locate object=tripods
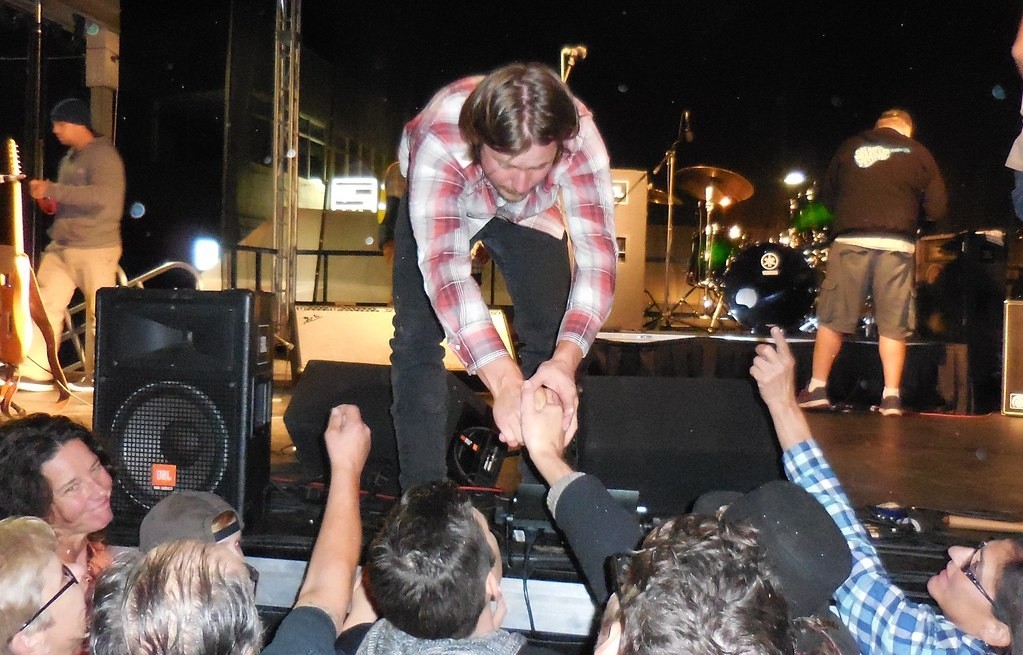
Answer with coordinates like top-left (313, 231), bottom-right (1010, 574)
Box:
top-left (641, 131), bottom-right (742, 337)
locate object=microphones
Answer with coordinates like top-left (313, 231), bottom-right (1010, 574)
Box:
top-left (562, 46), bottom-right (587, 61)
top-left (685, 112), bottom-right (694, 141)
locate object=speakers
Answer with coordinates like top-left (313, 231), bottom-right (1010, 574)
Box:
top-left (915, 231), bottom-right (1006, 415)
top-left (562, 374), bottom-right (787, 520)
top-left (284, 359), bottom-right (509, 498)
top-left (568, 168), bottom-right (647, 331)
top-left (1001, 298), bottom-right (1023, 417)
top-left (92, 287), bottom-right (279, 537)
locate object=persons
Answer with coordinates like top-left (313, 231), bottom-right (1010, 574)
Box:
top-left (0, 412), bottom-right (115, 573)
top-left (936, 336), bottom-right (970, 415)
top-left (520, 381), bottom-right (860, 655)
top-left (0, 515), bottom-right (88, 655)
top-left (334, 482), bottom-right (559, 655)
top-left (138, 488), bottom-right (244, 558)
top-left (593, 514), bottom-right (787, 655)
top-left (750, 327), bottom-right (1023, 655)
top-left (390, 64), bottom-right (618, 491)
top-left (0, 99), bottom-right (124, 392)
top-left (91, 403), bottom-right (371, 655)
top-left (1005, 21), bottom-right (1023, 223)
top-left (798, 110), bottom-right (947, 417)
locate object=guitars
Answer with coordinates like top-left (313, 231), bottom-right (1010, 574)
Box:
top-left (0, 137), bottom-right (30, 367)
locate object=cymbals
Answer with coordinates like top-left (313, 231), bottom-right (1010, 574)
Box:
top-left (647, 187), bottom-right (682, 205)
top-left (673, 166), bottom-right (754, 205)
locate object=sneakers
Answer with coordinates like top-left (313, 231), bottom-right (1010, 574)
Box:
top-left (879, 395), bottom-right (902, 416)
top-left (796, 383), bottom-right (830, 408)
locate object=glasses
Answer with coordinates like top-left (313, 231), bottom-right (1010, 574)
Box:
top-left (610, 552), bottom-right (639, 617)
top-left (959, 534), bottom-right (998, 611)
top-left (5, 564), bottom-right (80, 647)
top-left (243, 563), bottom-right (259, 599)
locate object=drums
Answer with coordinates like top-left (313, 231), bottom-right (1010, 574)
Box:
top-left (685, 224), bottom-right (752, 293)
top-left (723, 243), bottom-right (815, 335)
top-left (789, 187), bottom-right (833, 267)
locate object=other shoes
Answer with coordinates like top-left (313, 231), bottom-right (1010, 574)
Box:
top-left (9, 376), bottom-right (53, 391)
top-left (67, 377), bottom-right (95, 392)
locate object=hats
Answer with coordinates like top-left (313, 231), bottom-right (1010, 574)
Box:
top-left (51, 97), bottom-right (103, 138)
top-left (879, 108), bottom-right (912, 127)
top-left (693, 480), bottom-right (852, 619)
top-left (139, 489), bottom-right (243, 549)
